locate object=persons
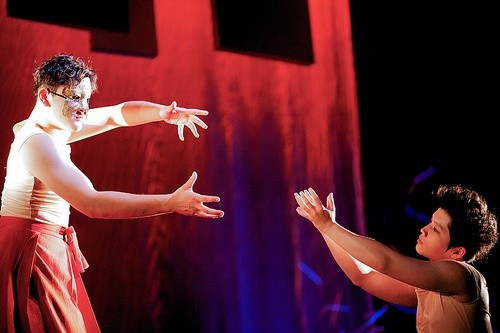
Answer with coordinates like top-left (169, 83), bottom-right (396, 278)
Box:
top-left (0, 52), bottom-right (225, 333)
top-left (295, 183), bottom-right (497, 333)
